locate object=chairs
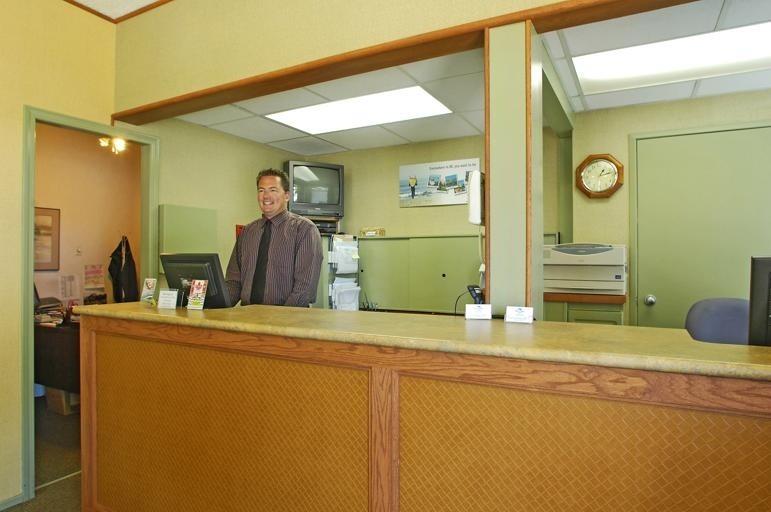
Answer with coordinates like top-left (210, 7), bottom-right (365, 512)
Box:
top-left (685, 296), bottom-right (750, 345)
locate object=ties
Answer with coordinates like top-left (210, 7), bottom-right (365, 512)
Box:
top-left (251, 219), bottom-right (272, 305)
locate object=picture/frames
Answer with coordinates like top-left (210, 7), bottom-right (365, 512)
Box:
top-left (34, 207), bottom-right (61, 272)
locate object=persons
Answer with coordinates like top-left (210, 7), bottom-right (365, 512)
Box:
top-left (225, 169), bottom-right (323, 307)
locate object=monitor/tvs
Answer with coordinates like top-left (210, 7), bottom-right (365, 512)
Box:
top-left (159, 252), bottom-right (231, 308)
top-left (283, 160), bottom-right (345, 217)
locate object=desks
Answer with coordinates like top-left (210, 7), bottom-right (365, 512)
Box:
top-left (34, 322), bottom-right (81, 417)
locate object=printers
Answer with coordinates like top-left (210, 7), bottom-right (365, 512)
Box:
top-left (543, 243), bottom-right (629, 295)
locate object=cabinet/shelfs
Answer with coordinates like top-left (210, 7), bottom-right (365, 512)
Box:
top-left (359, 234), bottom-right (484, 314)
top-left (544, 301), bottom-right (624, 325)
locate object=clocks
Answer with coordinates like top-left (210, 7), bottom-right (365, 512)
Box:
top-left (574, 153), bottom-right (625, 198)
